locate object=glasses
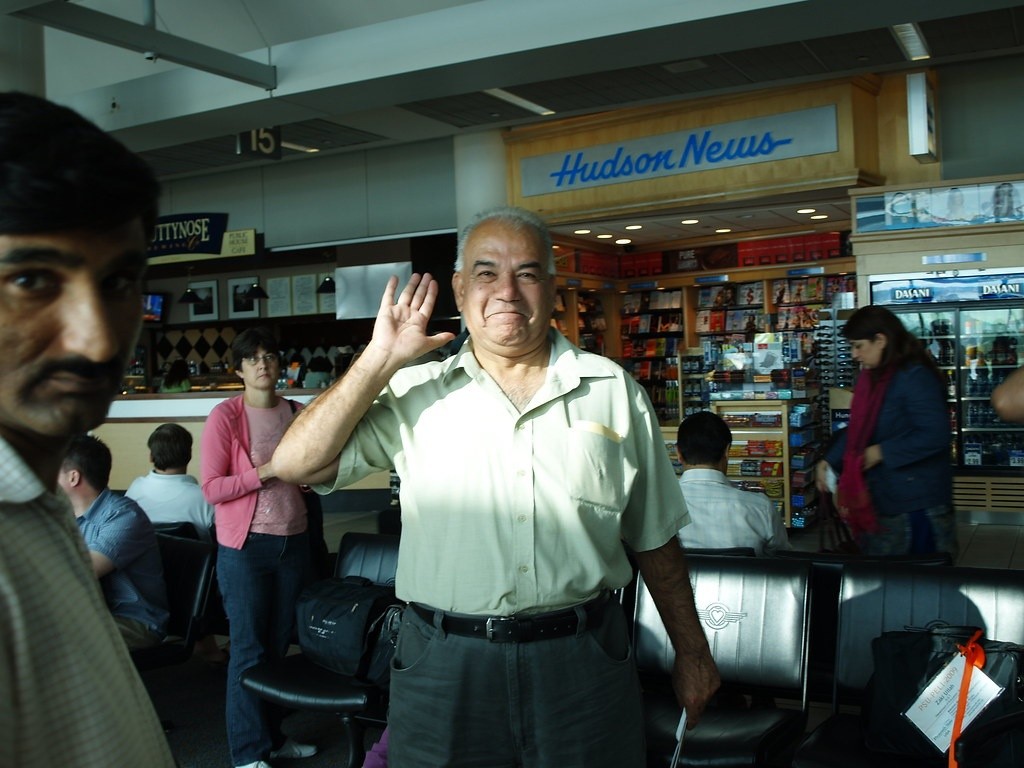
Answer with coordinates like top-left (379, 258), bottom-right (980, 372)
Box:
top-left (242, 353), bottom-right (274, 367)
top-left (814, 321), bottom-right (860, 451)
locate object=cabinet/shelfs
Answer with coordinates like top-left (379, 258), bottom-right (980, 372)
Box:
top-left (615, 282), bottom-right (683, 356)
top-left (552, 276), bottom-right (617, 356)
top-left (684, 272), bottom-right (851, 351)
top-left (707, 400), bottom-right (827, 530)
top-left (677, 345), bottom-right (724, 417)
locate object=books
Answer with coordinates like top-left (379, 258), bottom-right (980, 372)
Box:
top-left (550, 295), bottom-right (607, 351)
top-left (620, 275), bottom-right (857, 380)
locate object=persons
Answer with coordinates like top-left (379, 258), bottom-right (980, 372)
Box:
top-left (271, 206), bottom-right (721, 768)
top-left (124, 423), bottom-right (214, 529)
top-left (675, 410), bottom-right (792, 554)
top-left (795, 285), bottom-right (801, 301)
top-left (928, 319), bottom-right (969, 425)
top-left (783, 308), bottom-right (791, 329)
top-left (304, 356), bottom-right (330, 389)
top-left (159, 358), bottom-right (190, 392)
top-left (816, 303), bottom-right (959, 564)
top-left (59, 434), bottom-right (171, 668)
top-left (745, 328), bottom-right (759, 343)
top-left (776, 287), bottom-right (785, 302)
top-left (0, 91), bottom-right (177, 768)
top-left (745, 315), bottom-right (755, 329)
top-left (201, 330), bottom-right (317, 768)
top-left (991, 363), bottom-right (1024, 423)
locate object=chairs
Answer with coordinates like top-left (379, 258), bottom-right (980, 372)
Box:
top-left (150, 522), bottom-right (1024, 768)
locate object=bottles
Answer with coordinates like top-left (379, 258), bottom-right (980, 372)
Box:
top-left (950, 433), bottom-right (1021, 465)
top-left (781, 333), bottom-right (799, 362)
top-left (947, 369), bottom-right (1009, 428)
top-left (224, 358), bottom-right (229, 372)
top-left (189, 360), bottom-right (197, 375)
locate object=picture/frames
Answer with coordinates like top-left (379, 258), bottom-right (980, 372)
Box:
top-left (188, 280), bottom-right (219, 320)
top-left (228, 274), bottom-right (259, 318)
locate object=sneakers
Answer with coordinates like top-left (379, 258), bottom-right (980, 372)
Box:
top-left (235, 760), bottom-right (272, 768)
top-left (270, 737), bottom-right (317, 759)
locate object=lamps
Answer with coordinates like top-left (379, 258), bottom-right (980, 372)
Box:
top-left (180, 269), bottom-right (205, 304)
top-left (243, 260), bottom-right (268, 299)
top-left (314, 254), bottom-right (337, 294)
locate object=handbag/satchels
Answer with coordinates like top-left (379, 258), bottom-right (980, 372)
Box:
top-left (865, 620), bottom-right (1024, 767)
top-left (818, 489), bottom-right (858, 553)
top-left (296, 575), bottom-right (395, 678)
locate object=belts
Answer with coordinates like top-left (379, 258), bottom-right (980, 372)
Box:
top-left (410, 588), bottom-right (611, 645)
